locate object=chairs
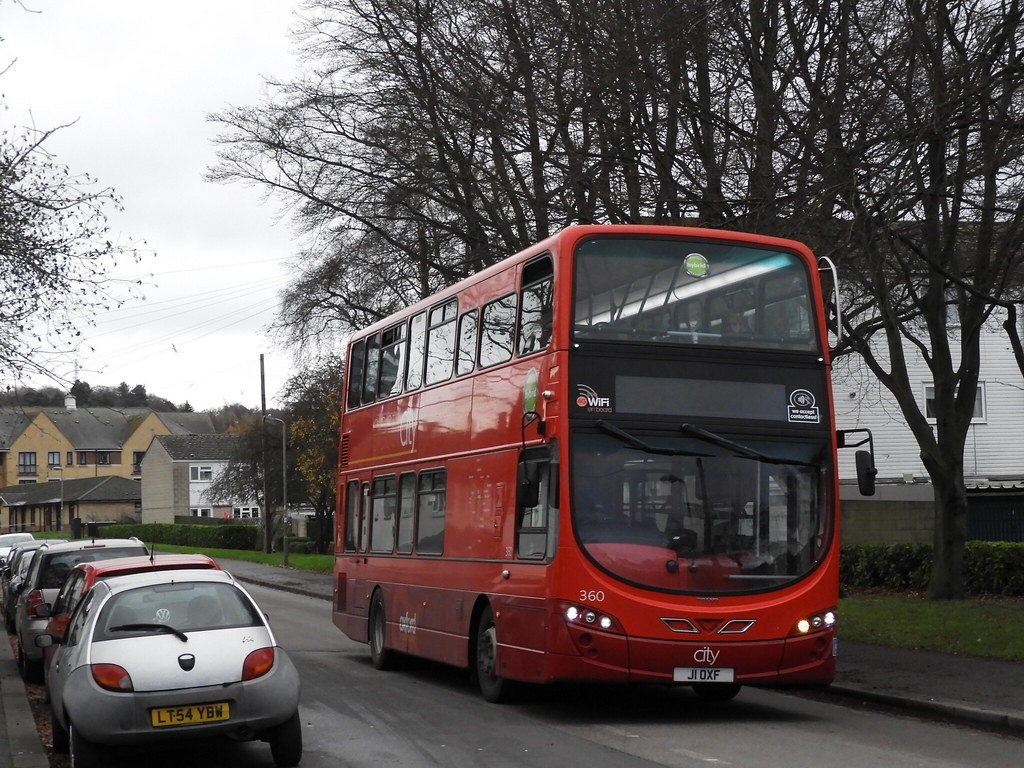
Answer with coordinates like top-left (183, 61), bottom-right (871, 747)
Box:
top-left (182, 596), bottom-right (220, 630)
top-left (109, 606), bottom-right (142, 636)
top-left (43, 562), bottom-right (70, 587)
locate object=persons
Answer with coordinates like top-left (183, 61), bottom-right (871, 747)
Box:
top-left (721, 314), bottom-right (753, 346)
top-left (570, 470), bottom-right (632, 526)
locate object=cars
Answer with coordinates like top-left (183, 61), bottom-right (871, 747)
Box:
top-left (34, 569), bottom-right (302, 768)
top-left (0, 532), bottom-right (222, 709)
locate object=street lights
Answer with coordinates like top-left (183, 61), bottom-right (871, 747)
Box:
top-left (53, 466), bottom-right (64, 539)
top-left (262, 414), bottom-right (289, 567)
